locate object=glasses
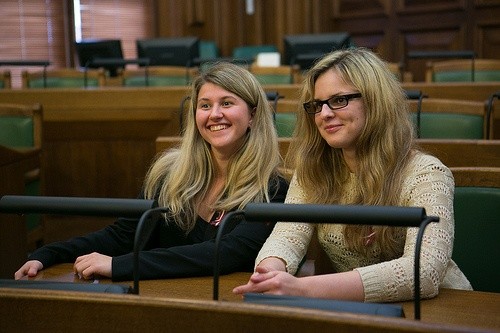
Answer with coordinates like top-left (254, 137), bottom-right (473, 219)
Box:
top-left (303, 93), bottom-right (361, 114)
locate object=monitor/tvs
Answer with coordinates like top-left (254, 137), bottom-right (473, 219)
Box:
top-left (283, 32), bottom-right (352, 73)
top-left (73, 39), bottom-right (126, 77)
top-left (136, 37), bottom-right (200, 69)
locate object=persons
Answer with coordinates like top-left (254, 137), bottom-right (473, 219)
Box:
top-left (15, 61), bottom-right (290, 283)
top-left (233, 46), bottom-right (473, 299)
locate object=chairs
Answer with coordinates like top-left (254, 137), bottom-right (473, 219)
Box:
top-left (0, 44), bottom-right (500, 292)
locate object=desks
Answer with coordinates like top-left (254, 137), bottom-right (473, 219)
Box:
top-left (0, 83), bottom-right (500, 333)
